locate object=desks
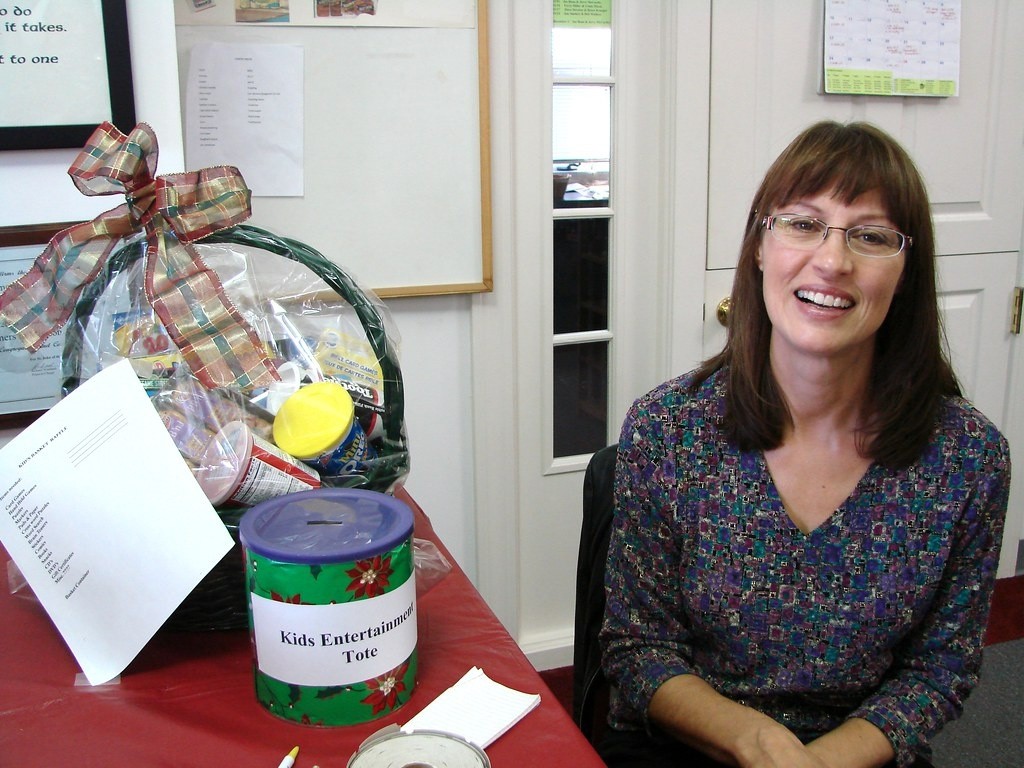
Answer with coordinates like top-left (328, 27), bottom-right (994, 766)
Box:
top-left (0, 482), bottom-right (608, 768)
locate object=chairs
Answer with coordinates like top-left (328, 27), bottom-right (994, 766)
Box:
top-left (572, 444), bottom-right (621, 768)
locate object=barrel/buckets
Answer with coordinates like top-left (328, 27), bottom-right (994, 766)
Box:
top-left (240, 486), bottom-right (418, 728)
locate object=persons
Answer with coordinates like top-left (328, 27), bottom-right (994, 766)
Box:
top-left (597, 120), bottom-right (1012, 768)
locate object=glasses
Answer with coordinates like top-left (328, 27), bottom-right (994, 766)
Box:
top-left (760, 212), bottom-right (914, 260)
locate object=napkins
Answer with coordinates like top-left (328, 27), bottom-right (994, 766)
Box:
top-left (400, 666), bottom-right (541, 750)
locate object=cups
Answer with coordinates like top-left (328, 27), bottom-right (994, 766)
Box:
top-left (265, 361), bottom-right (385, 440)
top-left (197, 420), bottom-right (320, 509)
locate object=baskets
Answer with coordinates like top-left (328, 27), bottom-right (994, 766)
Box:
top-left (60, 220), bottom-right (411, 634)
